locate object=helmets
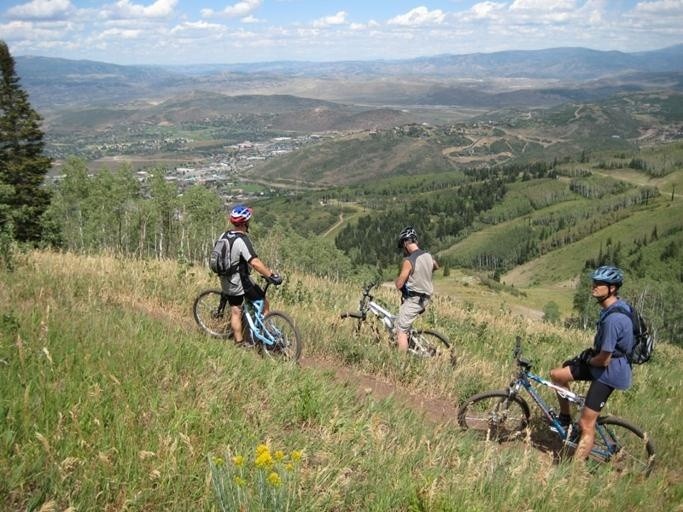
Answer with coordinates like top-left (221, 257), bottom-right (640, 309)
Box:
top-left (592, 266), bottom-right (625, 284)
top-left (398, 226), bottom-right (418, 248)
top-left (230, 207), bottom-right (253, 224)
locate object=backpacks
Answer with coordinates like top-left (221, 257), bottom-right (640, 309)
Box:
top-left (599, 305), bottom-right (655, 364)
top-left (210, 231), bottom-right (244, 275)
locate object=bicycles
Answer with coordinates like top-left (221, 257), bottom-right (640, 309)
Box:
top-left (335, 278), bottom-right (453, 366)
top-left (456, 335), bottom-right (655, 479)
top-left (193, 270), bottom-right (301, 364)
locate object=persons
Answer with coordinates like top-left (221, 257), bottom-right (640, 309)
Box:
top-left (396, 225), bottom-right (439, 353)
top-left (549, 266), bottom-right (634, 465)
top-left (218, 206), bottom-right (283, 348)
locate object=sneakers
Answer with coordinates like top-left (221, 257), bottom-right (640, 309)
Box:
top-left (235, 340), bottom-right (252, 348)
top-left (541, 413), bottom-right (571, 433)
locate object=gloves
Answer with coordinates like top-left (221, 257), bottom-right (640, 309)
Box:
top-left (269, 274), bottom-right (283, 285)
top-left (561, 349), bottom-right (595, 367)
